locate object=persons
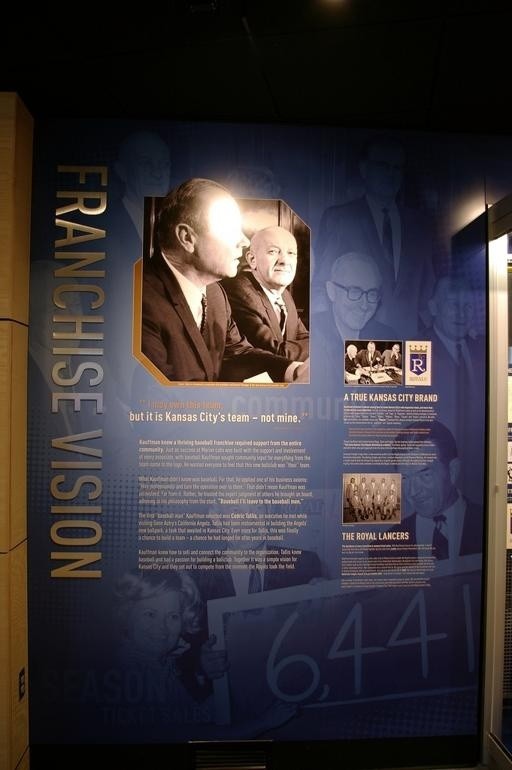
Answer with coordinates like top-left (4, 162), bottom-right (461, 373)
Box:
top-left (345, 341), bottom-right (401, 375)
top-left (345, 478), bottom-right (399, 520)
top-left (189, 479), bottom-right (321, 595)
top-left (117, 562), bottom-right (200, 659)
top-left (423, 273), bottom-right (488, 377)
top-left (143, 179), bottom-right (308, 383)
top-left (313, 252), bottom-right (399, 350)
top-left (368, 422), bottom-right (483, 566)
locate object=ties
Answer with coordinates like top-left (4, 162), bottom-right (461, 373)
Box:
top-left (276, 300), bottom-right (287, 333)
top-left (244, 558), bottom-right (262, 593)
top-left (200, 293), bottom-right (207, 337)
top-left (432, 514), bottom-right (449, 559)
top-left (332, 280), bottom-right (381, 303)
